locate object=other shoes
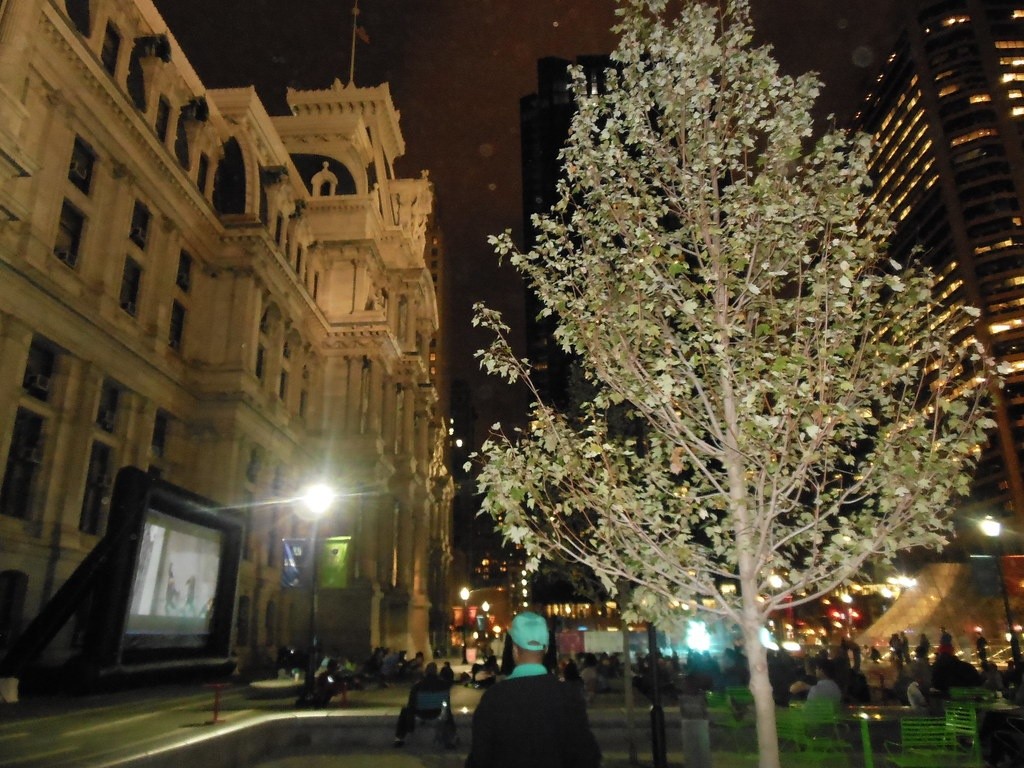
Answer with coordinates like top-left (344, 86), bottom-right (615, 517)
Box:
top-left (394, 741), bottom-right (403, 748)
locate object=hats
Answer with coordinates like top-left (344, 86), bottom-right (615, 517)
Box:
top-left (507, 611), bottom-right (550, 651)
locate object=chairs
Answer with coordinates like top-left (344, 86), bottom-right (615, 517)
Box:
top-left (705, 686), bottom-right (994, 768)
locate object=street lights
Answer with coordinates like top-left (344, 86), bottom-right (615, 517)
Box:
top-left (978, 512), bottom-right (1024, 670)
top-left (480, 600), bottom-right (490, 660)
top-left (460, 587), bottom-right (470, 665)
top-left (294, 479), bottom-right (335, 711)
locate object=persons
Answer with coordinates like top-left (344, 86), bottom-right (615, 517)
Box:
top-left (256, 641), bottom-right (502, 694)
top-left (552, 625), bottom-right (1024, 735)
top-left (467, 613), bottom-right (605, 767)
top-left (392, 662), bottom-right (458, 748)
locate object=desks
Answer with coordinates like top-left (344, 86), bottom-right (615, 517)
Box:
top-left (974, 703), bottom-right (1020, 732)
top-left (832, 712), bottom-right (896, 768)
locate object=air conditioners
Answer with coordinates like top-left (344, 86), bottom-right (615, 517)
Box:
top-left (33, 374), bottom-right (49, 394)
top-left (71, 161), bottom-right (88, 180)
top-left (95, 472), bottom-right (113, 488)
top-left (171, 340), bottom-right (181, 352)
top-left (122, 301), bottom-right (138, 315)
top-left (52, 249), bottom-right (72, 267)
top-left (102, 408), bottom-right (117, 424)
top-left (29, 443), bottom-right (41, 462)
top-left (135, 226), bottom-right (145, 245)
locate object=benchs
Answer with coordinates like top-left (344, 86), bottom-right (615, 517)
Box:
top-left (415, 702), bottom-right (448, 744)
top-left (249, 679), bottom-right (306, 697)
